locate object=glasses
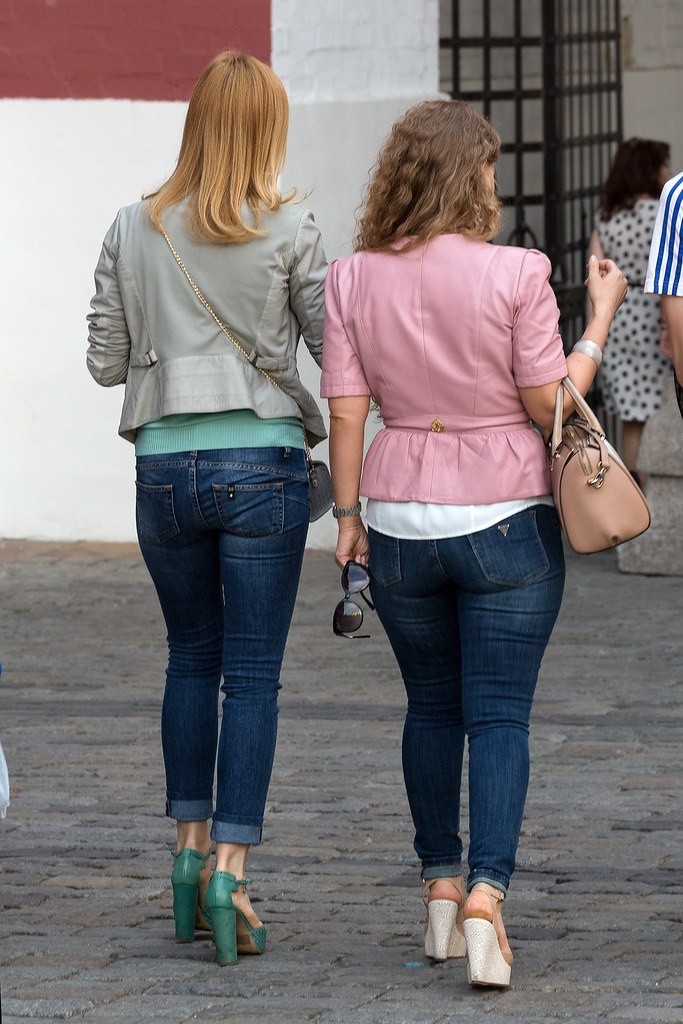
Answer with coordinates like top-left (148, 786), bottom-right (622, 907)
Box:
top-left (332, 560), bottom-right (375, 639)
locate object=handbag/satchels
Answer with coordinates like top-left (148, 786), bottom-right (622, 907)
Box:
top-left (548, 377), bottom-right (652, 555)
top-left (308, 460), bottom-right (333, 522)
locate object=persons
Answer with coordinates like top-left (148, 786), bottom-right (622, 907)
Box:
top-left (643, 170), bottom-right (683, 419)
top-left (319, 100), bottom-right (630, 988)
top-left (588, 137), bottom-right (671, 496)
top-left (86, 51), bottom-right (328, 966)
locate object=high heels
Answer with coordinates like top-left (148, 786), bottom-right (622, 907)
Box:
top-left (170, 847), bottom-right (212, 943)
top-left (204, 871), bottom-right (267, 966)
top-left (421, 876), bottom-right (467, 963)
top-left (462, 882), bottom-right (513, 987)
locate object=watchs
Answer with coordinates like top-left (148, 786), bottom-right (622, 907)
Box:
top-left (332, 501), bottom-right (364, 518)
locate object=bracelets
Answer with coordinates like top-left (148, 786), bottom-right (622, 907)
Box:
top-left (570, 340), bottom-right (603, 371)
